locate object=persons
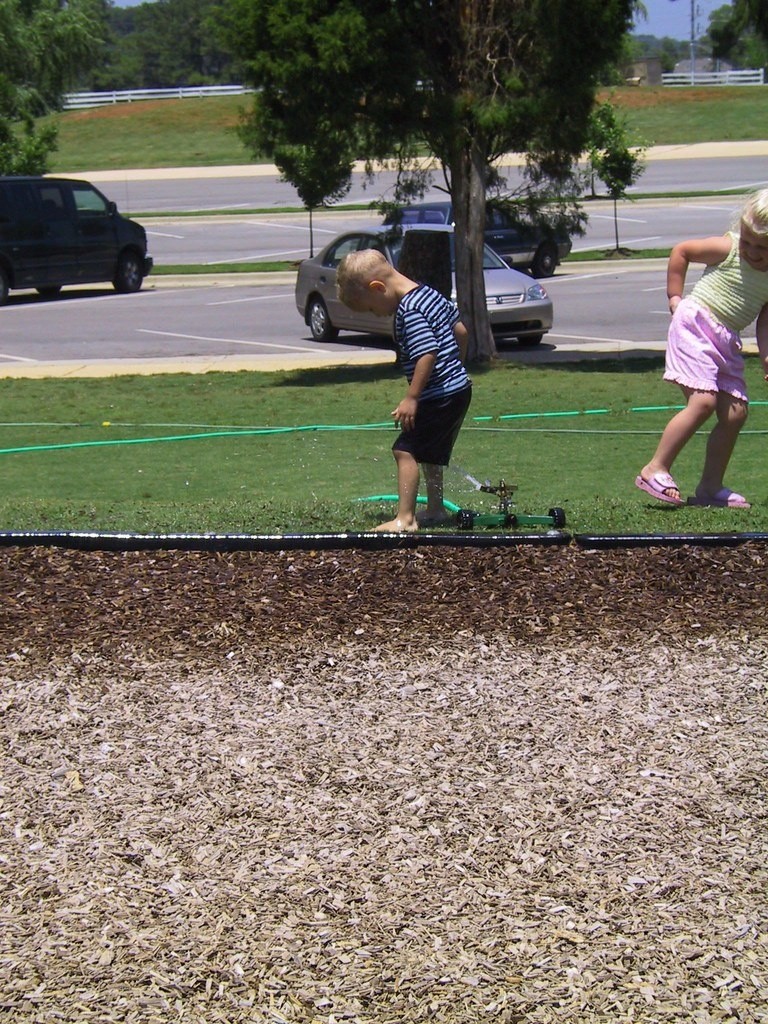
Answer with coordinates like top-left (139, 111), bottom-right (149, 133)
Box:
top-left (337, 248), bottom-right (474, 532)
top-left (635, 189), bottom-right (768, 510)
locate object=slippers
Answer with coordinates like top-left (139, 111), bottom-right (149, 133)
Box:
top-left (688, 488), bottom-right (750, 508)
top-left (635, 472), bottom-right (685, 506)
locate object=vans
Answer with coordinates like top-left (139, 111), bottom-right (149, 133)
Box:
top-left (0, 177), bottom-right (153, 304)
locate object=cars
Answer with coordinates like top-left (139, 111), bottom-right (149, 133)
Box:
top-left (297, 223), bottom-right (553, 351)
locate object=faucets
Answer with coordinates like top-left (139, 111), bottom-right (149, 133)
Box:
top-left (478, 476), bottom-right (519, 514)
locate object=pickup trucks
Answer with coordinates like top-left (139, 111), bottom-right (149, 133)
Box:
top-left (382, 200), bottom-right (572, 276)
